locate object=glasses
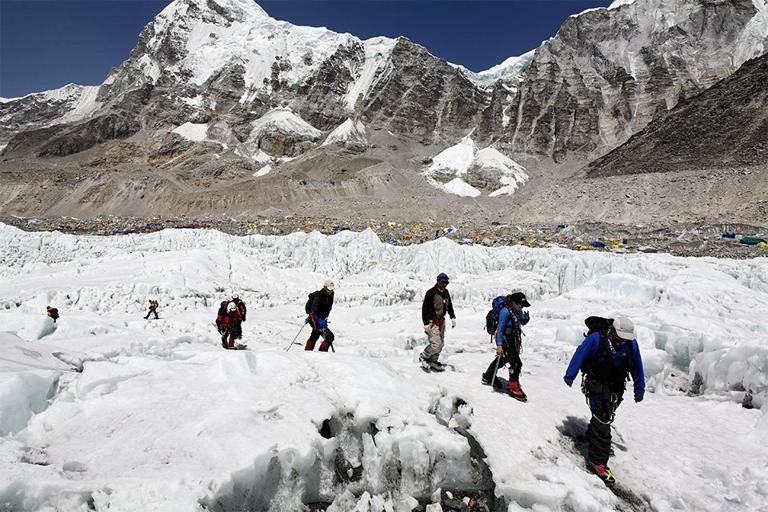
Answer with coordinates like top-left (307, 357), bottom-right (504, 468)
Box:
top-left (441, 281), bottom-right (449, 283)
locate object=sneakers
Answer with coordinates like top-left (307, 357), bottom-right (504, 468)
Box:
top-left (481, 373), bottom-right (503, 388)
top-left (431, 362), bottom-right (445, 371)
top-left (589, 460), bottom-right (615, 482)
top-left (508, 381), bottom-right (526, 401)
top-left (419, 353), bottom-right (430, 369)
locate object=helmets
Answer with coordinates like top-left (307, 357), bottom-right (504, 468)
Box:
top-left (227, 302), bottom-right (237, 313)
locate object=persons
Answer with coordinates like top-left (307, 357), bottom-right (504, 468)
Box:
top-left (420, 272), bottom-right (456, 371)
top-left (563, 317), bottom-right (645, 476)
top-left (216, 301), bottom-right (241, 349)
top-left (144, 300), bottom-right (158, 319)
top-left (231, 294), bottom-right (247, 339)
top-left (484, 292), bottom-right (531, 400)
top-left (47, 306), bottom-right (56, 322)
top-left (305, 280), bottom-right (335, 351)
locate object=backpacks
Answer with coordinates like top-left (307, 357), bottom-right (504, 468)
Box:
top-left (305, 291), bottom-right (318, 313)
top-left (486, 309), bottom-right (498, 343)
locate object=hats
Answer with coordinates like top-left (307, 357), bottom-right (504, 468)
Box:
top-left (511, 293), bottom-right (530, 307)
top-left (325, 280), bottom-right (335, 291)
top-left (437, 273), bottom-right (449, 280)
top-left (613, 317), bottom-right (634, 341)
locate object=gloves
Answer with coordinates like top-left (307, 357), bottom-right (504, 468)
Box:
top-left (451, 319), bottom-right (456, 328)
top-left (524, 310), bottom-right (529, 320)
top-left (424, 325), bottom-right (431, 336)
top-left (634, 393), bottom-right (643, 402)
top-left (318, 319), bottom-right (326, 326)
